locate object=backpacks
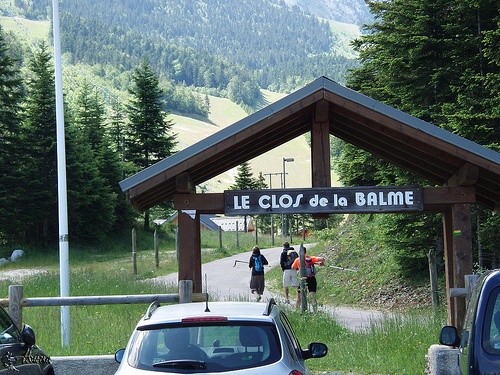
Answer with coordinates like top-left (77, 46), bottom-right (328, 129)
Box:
top-left (282, 249), bottom-right (298, 268)
top-left (250, 255), bottom-right (264, 272)
top-left (304, 256), bottom-right (315, 277)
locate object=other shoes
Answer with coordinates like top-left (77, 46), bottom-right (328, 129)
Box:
top-left (256, 293), bottom-right (262, 302)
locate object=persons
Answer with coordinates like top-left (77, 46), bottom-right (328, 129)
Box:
top-left (291, 247), bottom-right (324, 312)
top-left (280, 242), bottom-right (300, 303)
top-left (249, 246), bottom-right (268, 302)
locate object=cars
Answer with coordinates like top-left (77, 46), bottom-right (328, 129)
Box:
top-left (0, 306), bottom-right (57, 375)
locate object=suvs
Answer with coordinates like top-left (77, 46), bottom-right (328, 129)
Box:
top-left (438, 269), bottom-right (500, 375)
top-left (114, 298), bottom-right (328, 375)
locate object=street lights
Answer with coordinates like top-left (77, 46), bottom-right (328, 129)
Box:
top-left (282, 157), bottom-right (295, 237)
